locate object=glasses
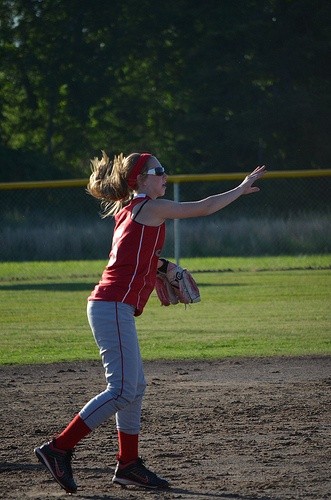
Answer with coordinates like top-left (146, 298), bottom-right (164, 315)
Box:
top-left (143, 167), bottom-right (164, 176)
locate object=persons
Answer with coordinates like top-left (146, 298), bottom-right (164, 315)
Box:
top-left (33, 151), bottom-right (268, 494)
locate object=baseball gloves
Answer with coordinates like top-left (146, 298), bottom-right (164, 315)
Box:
top-left (155, 259), bottom-right (201, 306)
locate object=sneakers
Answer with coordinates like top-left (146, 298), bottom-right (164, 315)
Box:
top-left (112, 456), bottom-right (169, 489)
top-left (34, 437), bottom-right (77, 493)
